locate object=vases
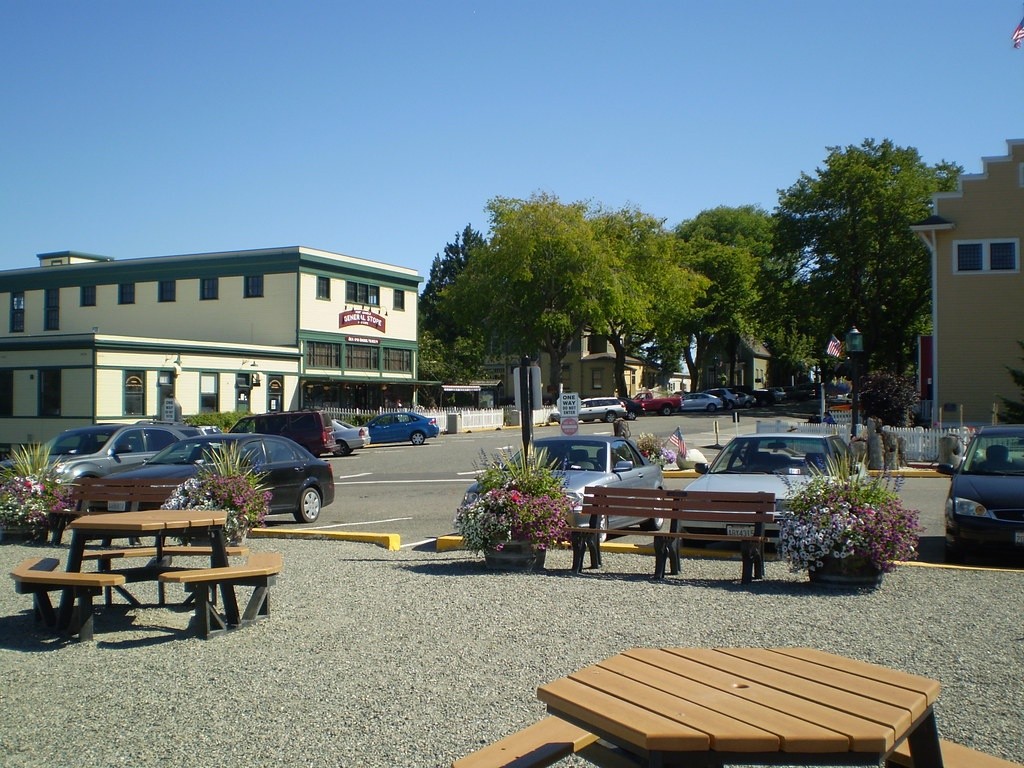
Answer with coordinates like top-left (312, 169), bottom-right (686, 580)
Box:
top-left (196, 527), bottom-right (247, 546)
top-left (808, 557), bottom-right (881, 588)
top-left (1, 525), bottom-right (46, 536)
top-left (484, 539), bottom-right (546, 570)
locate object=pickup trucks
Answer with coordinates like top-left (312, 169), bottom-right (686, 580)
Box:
top-left (629, 391), bottom-right (683, 415)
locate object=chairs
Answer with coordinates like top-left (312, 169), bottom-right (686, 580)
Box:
top-left (597, 449), bottom-right (614, 471)
top-left (973, 445), bottom-right (1019, 472)
top-left (744, 452), bottom-right (773, 474)
top-left (189, 445), bottom-right (212, 463)
top-left (803, 452), bottom-right (826, 470)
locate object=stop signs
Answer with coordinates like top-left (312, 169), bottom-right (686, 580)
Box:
top-left (560, 418), bottom-right (579, 436)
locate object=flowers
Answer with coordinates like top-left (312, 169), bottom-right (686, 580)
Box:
top-left (772, 448), bottom-right (928, 575)
top-left (635, 432), bottom-right (676, 470)
top-left (0, 439), bottom-right (76, 531)
top-left (159, 439), bottom-right (274, 548)
top-left (450, 441), bottom-right (580, 561)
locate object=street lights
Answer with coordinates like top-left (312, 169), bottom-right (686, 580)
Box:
top-left (846, 326), bottom-right (864, 440)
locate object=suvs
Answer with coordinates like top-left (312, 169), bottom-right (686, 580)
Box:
top-left (228, 409), bottom-right (338, 458)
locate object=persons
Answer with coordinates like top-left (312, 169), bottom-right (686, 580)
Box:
top-left (823, 412), bottom-right (833, 424)
top-left (808, 413), bottom-right (820, 423)
top-left (397, 399), bottom-right (402, 408)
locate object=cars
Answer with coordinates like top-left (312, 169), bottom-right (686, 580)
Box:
top-left (681, 392), bottom-right (724, 412)
top-left (331, 419), bottom-right (371, 457)
top-left (701, 385), bottom-right (818, 410)
top-left (460, 436), bottom-right (664, 543)
top-left (936, 424), bottom-right (1024, 554)
top-left (0, 421), bottom-right (224, 483)
top-left (89, 433), bottom-right (335, 523)
top-left (618, 397), bottom-right (646, 420)
top-left (578, 396), bottom-right (628, 423)
top-left (677, 432), bottom-right (868, 549)
top-left (362, 412), bottom-right (439, 445)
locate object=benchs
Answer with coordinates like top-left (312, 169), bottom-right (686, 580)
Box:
top-left (80, 547), bottom-right (249, 607)
top-left (45, 478), bottom-right (192, 547)
top-left (571, 449), bottom-right (596, 469)
top-left (451, 713), bottom-right (600, 768)
top-left (10, 557), bottom-right (126, 644)
top-left (157, 553), bottom-right (282, 642)
top-left (883, 731), bottom-right (1024, 768)
top-left (561, 485), bottom-right (777, 587)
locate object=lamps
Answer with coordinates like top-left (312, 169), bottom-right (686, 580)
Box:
top-left (174, 358), bottom-right (182, 363)
top-left (251, 362), bottom-right (258, 366)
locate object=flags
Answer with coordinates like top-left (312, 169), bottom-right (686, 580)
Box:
top-left (669, 429), bottom-right (687, 457)
top-left (827, 337), bottom-right (842, 357)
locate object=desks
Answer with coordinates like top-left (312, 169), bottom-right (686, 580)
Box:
top-left (534, 648), bottom-right (945, 768)
top-left (56, 509), bottom-right (241, 635)
